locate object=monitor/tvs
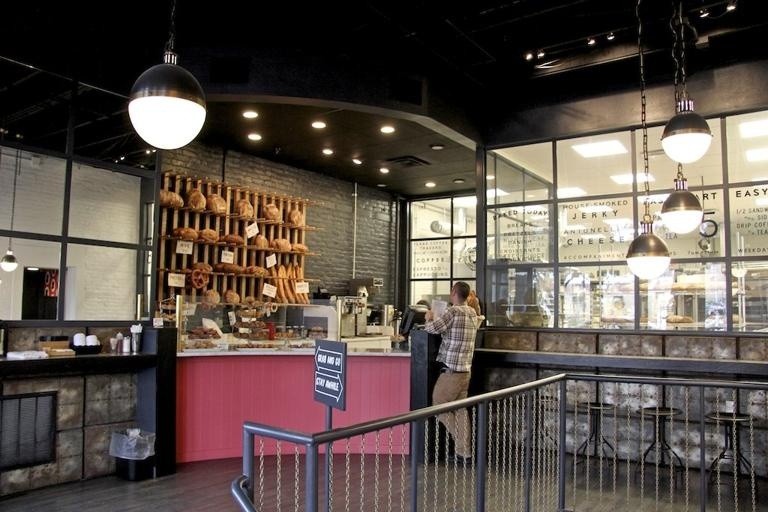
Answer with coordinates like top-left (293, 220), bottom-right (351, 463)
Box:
top-left (400, 304), bottom-right (428, 335)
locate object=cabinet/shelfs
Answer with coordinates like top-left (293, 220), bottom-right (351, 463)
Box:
top-left (155, 167), bottom-right (322, 321)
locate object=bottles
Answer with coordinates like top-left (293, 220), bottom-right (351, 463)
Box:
top-left (110, 331), bottom-right (143, 353)
top-left (392, 311), bottom-right (402, 336)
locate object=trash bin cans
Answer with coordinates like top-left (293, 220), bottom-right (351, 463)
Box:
top-left (111, 428), bottom-right (156, 481)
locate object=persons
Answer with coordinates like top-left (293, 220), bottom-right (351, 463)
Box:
top-left (522, 286), bottom-right (549, 328)
top-left (424, 282), bottom-right (476, 465)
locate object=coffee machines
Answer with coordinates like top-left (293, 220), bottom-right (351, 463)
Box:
top-left (344, 276), bottom-right (395, 336)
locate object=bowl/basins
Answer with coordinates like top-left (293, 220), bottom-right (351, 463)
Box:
top-left (70, 345), bottom-right (103, 355)
top-left (73, 332), bottom-right (100, 346)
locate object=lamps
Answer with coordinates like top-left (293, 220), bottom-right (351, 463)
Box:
top-left (127, 1), bottom-right (207, 150)
top-left (626, 0), bottom-right (711, 278)
top-left (0, 137), bottom-right (19, 271)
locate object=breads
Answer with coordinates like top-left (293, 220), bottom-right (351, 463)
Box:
top-left (157, 186), bottom-right (324, 351)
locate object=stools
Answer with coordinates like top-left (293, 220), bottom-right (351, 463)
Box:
top-left (704, 413), bottom-right (758, 495)
top-left (518, 395), bottom-right (558, 458)
top-left (634, 407), bottom-right (684, 488)
top-left (572, 402), bottom-right (618, 468)
